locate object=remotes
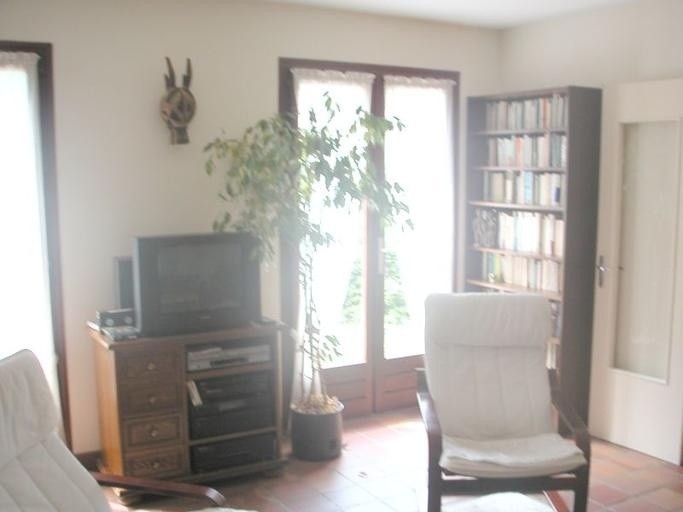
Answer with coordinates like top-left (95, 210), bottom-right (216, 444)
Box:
top-left (101, 326), bottom-right (123, 342)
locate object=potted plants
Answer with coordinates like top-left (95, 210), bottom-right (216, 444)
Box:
top-left (203, 104), bottom-right (417, 474)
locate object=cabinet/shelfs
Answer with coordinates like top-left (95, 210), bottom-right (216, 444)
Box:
top-left (460, 84), bottom-right (606, 443)
top-left (82, 318), bottom-right (292, 502)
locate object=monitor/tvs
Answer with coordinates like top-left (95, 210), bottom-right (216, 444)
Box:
top-left (132, 231), bottom-right (263, 334)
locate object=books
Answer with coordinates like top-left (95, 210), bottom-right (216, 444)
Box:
top-left (186, 380), bottom-right (204, 407)
top-left (467, 91), bottom-right (567, 370)
top-left (187, 346), bottom-right (222, 357)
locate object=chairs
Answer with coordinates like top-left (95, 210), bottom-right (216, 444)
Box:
top-left (0, 348), bottom-right (262, 511)
top-left (416, 288), bottom-right (595, 512)
